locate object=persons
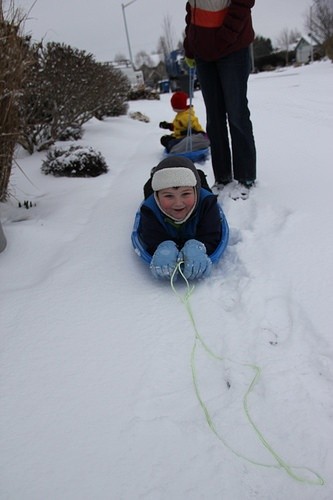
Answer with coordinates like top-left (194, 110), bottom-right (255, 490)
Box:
top-left (137, 155), bottom-right (222, 280)
top-left (159, 70), bottom-right (204, 153)
top-left (183, 0), bottom-right (257, 199)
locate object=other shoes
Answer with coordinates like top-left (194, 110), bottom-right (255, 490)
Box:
top-left (231, 180), bottom-right (254, 199)
top-left (210, 179), bottom-right (231, 193)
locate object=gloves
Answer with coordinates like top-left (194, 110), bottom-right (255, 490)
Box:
top-left (150, 240), bottom-right (180, 282)
top-left (159, 121), bottom-right (169, 129)
top-left (180, 239), bottom-right (212, 282)
top-left (185, 57), bottom-right (197, 68)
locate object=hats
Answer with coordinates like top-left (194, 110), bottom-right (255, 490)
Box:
top-left (171, 91), bottom-right (189, 111)
top-left (150, 157), bottom-right (201, 225)
